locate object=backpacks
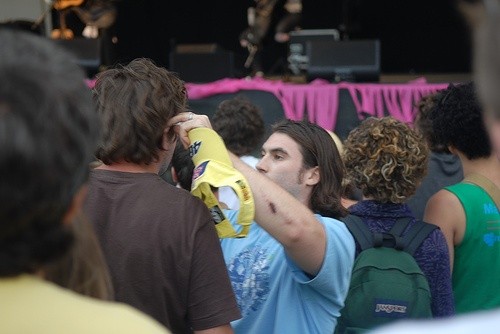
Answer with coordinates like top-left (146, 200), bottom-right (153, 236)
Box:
top-left (333, 214), bottom-right (441, 334)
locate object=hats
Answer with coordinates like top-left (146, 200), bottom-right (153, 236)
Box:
top-left (188, 128), bottom-right (255, 239)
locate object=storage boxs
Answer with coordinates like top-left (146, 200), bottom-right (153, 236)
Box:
top-left (288, 28), bottom-right (344, 71)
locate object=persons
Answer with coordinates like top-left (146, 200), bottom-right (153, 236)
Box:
top-left (0, 0), bottom-right (500, 334)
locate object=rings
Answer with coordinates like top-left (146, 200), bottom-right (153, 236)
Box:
top-left (188, 113), bottom-right (193, 120)
